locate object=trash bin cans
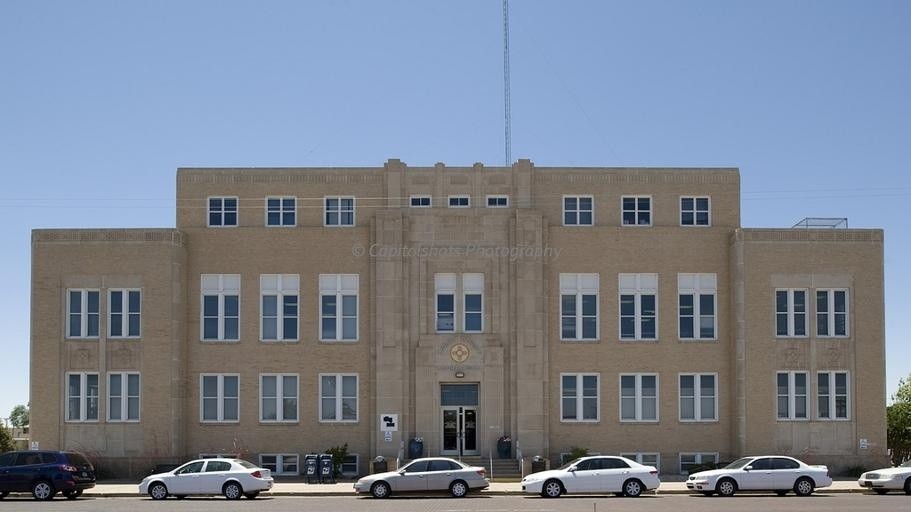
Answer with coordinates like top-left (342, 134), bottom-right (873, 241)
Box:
top-left (409, 438), bottom-right (423, 459)
top-left (373, 459), bottom-right (387, 473)
top-left (496, 437), bottom-right (511, 459)
top-left (529, 455), bottom-right (547, 472)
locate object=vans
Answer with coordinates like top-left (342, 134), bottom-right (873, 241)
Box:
top-left (0, 451), bottom-right (97, 501)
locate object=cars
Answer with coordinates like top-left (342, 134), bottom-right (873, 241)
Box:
top-left (138, 458), bottom-right (272, 500)
top-left (520, 456), bottom-right (660, 498)
top-left (686, 455), bottom-right (832, 497)
top-left (857, 460), bottom-right (911, 495)
top-left (353, 457), bottom-right (490, 498)
